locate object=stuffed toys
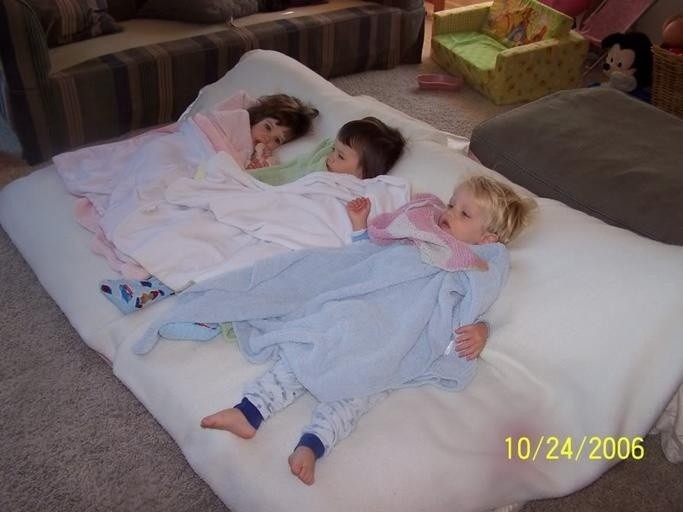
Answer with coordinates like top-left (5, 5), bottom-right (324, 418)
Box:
top-left (585, 31), bottom-right (653, 104)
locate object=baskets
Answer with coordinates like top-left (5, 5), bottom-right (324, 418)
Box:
top-left (651, 43), bottom-right (683, 118)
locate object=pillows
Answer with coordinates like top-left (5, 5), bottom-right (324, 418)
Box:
top-left (33, 1), bottom-right (125, 47)
top-left (467, 83), bottom-right (682, 255)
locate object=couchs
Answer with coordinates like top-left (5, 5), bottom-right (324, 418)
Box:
top-left (0, 0), bottom-right (426, 165)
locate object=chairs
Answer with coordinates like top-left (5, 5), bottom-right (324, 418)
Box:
top-left (429, 2), bottom-right (593, 106)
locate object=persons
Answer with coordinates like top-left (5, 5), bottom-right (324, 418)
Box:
top-left (202, 173), bottom-right (532, 486)
top-left (248, 93), bottom-right (319, 168)
top-left (99, 116), bottom-right (406, 314)
